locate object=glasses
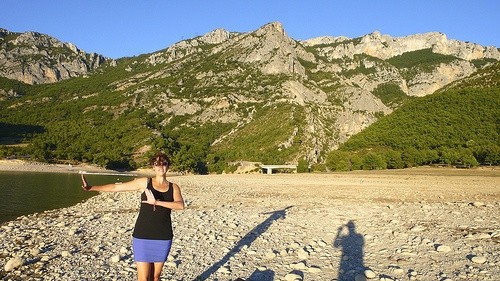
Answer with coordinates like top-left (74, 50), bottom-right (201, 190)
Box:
top-left (155, 162), bottom-right (167, 166)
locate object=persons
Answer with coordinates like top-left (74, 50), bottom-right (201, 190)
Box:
top-left (79, 152), bottom-right (184, 280)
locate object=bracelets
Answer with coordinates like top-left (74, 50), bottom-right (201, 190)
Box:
top-left (152, 198), bottom-right (160, 211)
